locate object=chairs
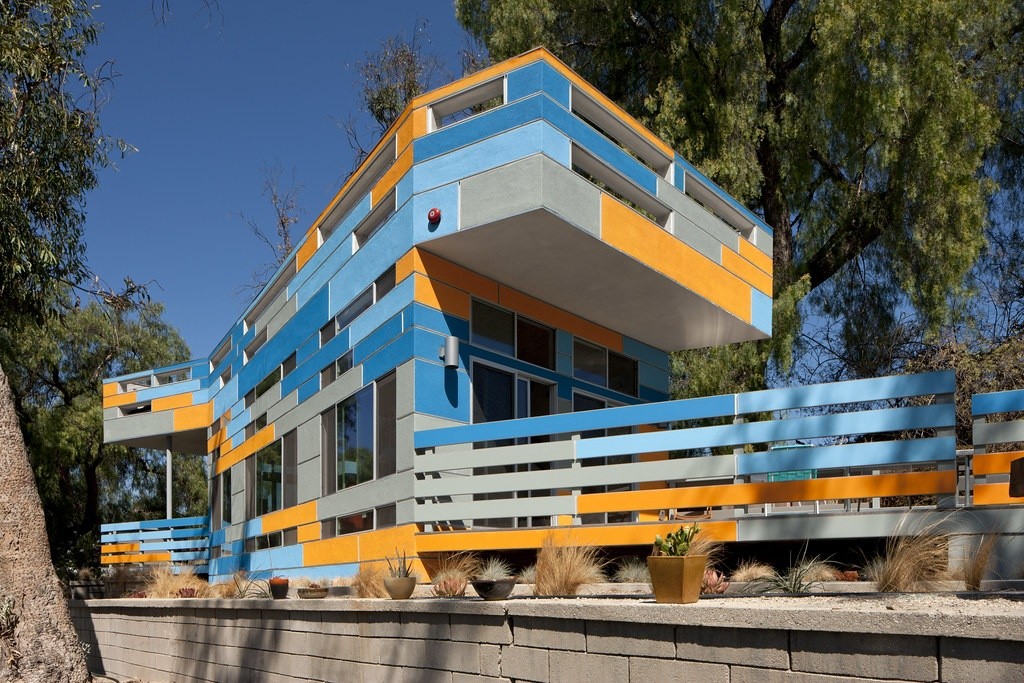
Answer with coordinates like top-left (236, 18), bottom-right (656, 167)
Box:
top-left (761, 444), bottom-right (820, 517)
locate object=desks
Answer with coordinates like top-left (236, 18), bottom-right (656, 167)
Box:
top-left (842, 448), bottom-right (975, 511)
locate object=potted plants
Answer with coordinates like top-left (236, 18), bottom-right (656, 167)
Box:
top-left (383, 545), bottom-right (417, 600)
top-left (269, 576), bottom-right (289, 599)
top-left (471, 554), bottom-right (519, 601)
top-left (296, 581), bottom-right (329, 599)
top-left (328, 577), bottom-right (354, 598)
top-left (646, 519), bottom-right (708, 604)
